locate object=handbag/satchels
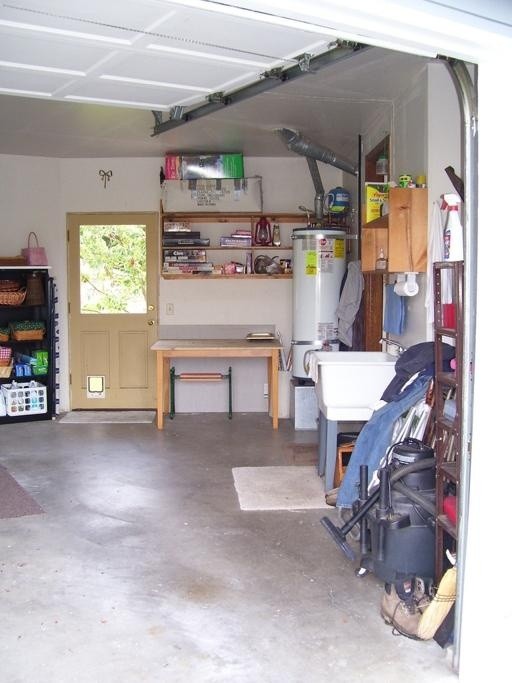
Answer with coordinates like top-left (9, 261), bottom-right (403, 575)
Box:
top-left (21, 232), bottom-right (48, 266)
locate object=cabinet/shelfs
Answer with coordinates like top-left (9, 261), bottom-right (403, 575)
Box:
top-left (431, 259), bottom-right (463, 653)
top-left (159, 209), bottom-right (345, 279)
top-left (361, 186), bottom-right (428, 274)
top-left (0, 267), bottom-right (57, 423)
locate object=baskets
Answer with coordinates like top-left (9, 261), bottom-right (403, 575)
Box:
top-left (9, 323), bottom-right (45, 341)
top-left (0, 358), bottom-right (14, 378)
top-left (0, 287), bottom-right (27, 306)
top-left (1, 382), bottom-right (47, 417)
top-left (0, 334), bottom-right (9, 341)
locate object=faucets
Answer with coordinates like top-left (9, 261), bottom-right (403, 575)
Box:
top-left (376, 338), bottom-right (407, 352)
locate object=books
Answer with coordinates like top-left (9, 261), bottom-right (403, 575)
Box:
top-left (365, 183), bottom-right (389, 223)
top-left (162, 218), bottom-right (254, 276)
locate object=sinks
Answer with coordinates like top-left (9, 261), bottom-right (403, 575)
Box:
top-left (309, 350), bottom-right (399, 421)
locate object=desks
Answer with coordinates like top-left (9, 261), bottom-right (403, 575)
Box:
top-left (149, 336), bottom-right (286, 430)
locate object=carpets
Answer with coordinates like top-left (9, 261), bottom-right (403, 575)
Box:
top-left (59, 410), bottom-right (156, 426)
top-left (1, 461), bottom-right (45, 521)
top-left (229, 460), bottom-right (336, 512)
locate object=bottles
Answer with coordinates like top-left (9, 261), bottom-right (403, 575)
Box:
top-left (375, 155), bottom-right (389, 181)
top-left (272, 224), bottom-right (280, 246)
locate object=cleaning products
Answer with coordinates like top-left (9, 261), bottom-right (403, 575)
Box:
top-left (440, 193), bottom-right (464, 265)
